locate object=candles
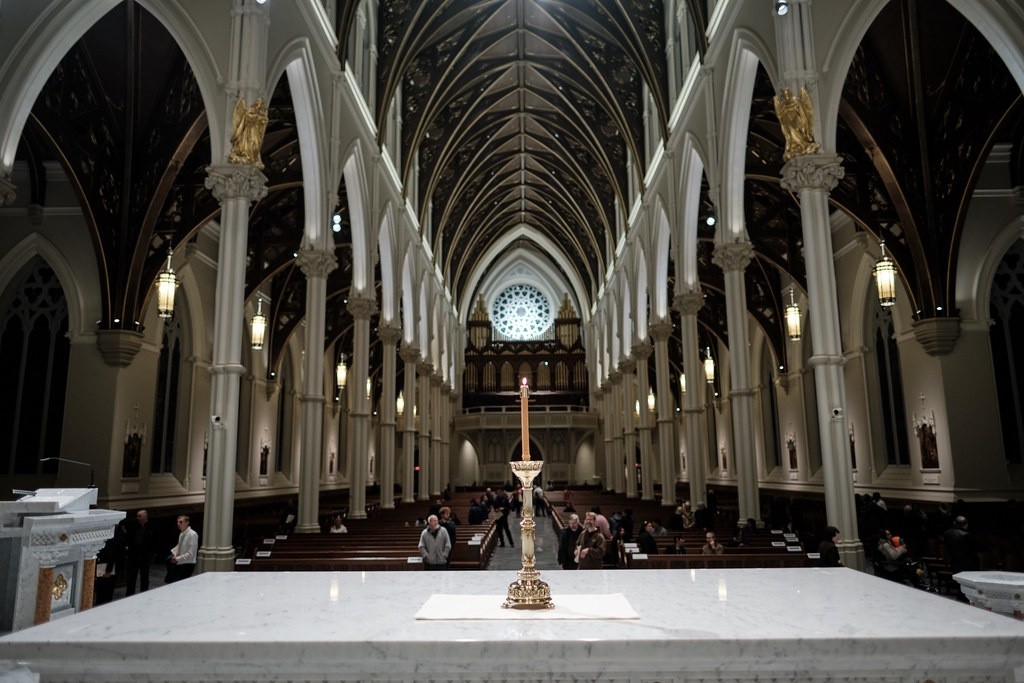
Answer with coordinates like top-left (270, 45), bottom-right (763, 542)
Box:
top-left (519, 377), bottom-right (531, 460)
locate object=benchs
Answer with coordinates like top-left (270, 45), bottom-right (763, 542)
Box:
top-left (232, 490), bottom-right (518, 571)
top-left (542, 490), bottom-right (824, 569)
top-left (921, 556), bottom-right (955, 594)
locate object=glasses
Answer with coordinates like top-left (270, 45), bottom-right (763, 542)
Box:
top-left (176, 521), bottom-right (183, 524)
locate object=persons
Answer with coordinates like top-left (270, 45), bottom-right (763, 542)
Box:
top-left (773, 87), bottom-right (821, 162)
top-left (95, 510), bottom-right (199, 604)
top-left (559, 488), bottom-right (977, 604)
top-left (330, 515), bottom-right (347, 533)
top-left (279, 499), bottom-right (297, 532)
top-left (227, 96), bottom-right (269, 164)
top-left (414, 479), bottom-right (555, 571)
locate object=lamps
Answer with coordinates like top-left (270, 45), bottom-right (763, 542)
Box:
top-left (635, 401), bottom-right (641, 415)
top-left (647, 386), bottom-right (656, 412)
top-left (155, 250), bottom-right (178, 317)
top-left (912, 307), bottom-right (961, 321)
top-left (96, 319), bottom-right (140, 331)
top-left (706, 214), bottom-right (717, 226)
top-left (775, 0), bottom-right (788, 15)
top-left (873, 240), bottom-right (897, 305)
top-left (251, 285), bottom-right (267, 349)
top-left (679, 374), bottom-right (686, 393)
top-left (396, 390), bottom-right (405, 415)
top-left (413, 405), bottom-right (417, 416)
top-left (784, 278), bottom-right (802, 340)
top-left (703, 347), bottom-right (715, 383)
top-left (337, 353), bottom-right (348, 388)
top-left (270, 371), bottom-right (276, 379)
top-left (779, 366), bottom-right (785, 373)
top-left (366, 377), bottom-right (371, 399)
